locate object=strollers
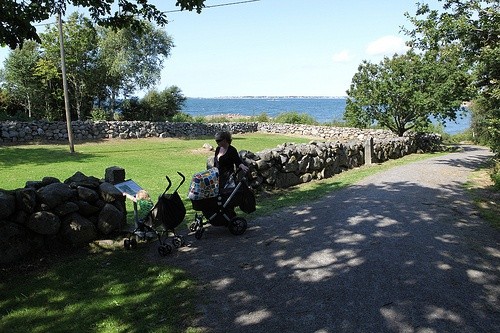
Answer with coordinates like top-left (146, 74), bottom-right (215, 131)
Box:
top-left (114, 172), bottom-right (186, 256)
top-left (188, 165), bottom-right (250, 242)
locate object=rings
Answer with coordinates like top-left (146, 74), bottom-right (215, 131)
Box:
top-left (244, 168), bottom-right (246, 171)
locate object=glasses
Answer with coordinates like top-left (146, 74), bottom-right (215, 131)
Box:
top-left (216, 139), bottom-right (223, 142)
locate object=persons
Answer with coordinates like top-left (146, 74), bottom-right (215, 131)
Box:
top-left (213, 130), bottom-right (250, 219)
top-left (122, 190), bottom-right (154, 238)
